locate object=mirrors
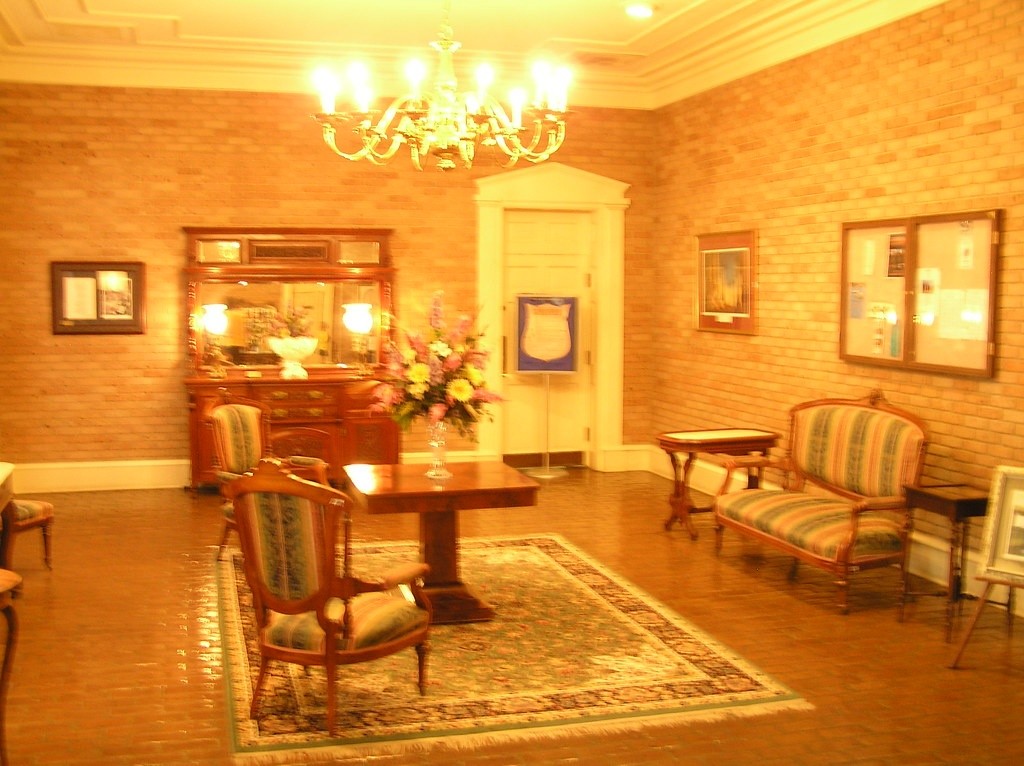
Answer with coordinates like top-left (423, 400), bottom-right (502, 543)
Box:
top-left (189, 278), bottom-right (390, 375)
top-left (838, 208), bottom-right (1000, 377)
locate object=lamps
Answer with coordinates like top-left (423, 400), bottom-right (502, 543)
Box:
top-left (311, 19), bottom-right (580, 175)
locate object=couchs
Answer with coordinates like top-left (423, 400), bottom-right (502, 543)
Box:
top-left (713, 388), bottom-right (929, 616)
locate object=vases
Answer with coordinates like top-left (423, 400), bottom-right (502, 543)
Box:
top-left (268, 335), bottom-right (318, 379)
top-left (424, 423), bottom-right (454, 479)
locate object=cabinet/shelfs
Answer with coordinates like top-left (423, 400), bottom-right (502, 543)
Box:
top-left (898, 482), bottom-right (991, 644)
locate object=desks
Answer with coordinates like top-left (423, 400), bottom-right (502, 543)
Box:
top-left (656, 428), bottom-right (781, 540)
top-left (343, 459), bottom-right (541, 626)
top-left (184, 372), bottom-right (400, 496)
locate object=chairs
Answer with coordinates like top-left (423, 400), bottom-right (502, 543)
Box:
top-left (203, 395), bottom-right (332, 562)
top-left (0, 463), bottom-right (57, 766)
top-left (225, 458), bottom-right (432, 737)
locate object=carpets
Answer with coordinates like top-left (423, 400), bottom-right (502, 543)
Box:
top-left (218, 531), bottom-right (818, 766)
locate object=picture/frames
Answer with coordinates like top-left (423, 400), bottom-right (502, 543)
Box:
top-left (696, 229), bottom-right (758, 336)
top-left (975, 465), bottom-right (1024, 587)
top-left (50, 262), bottom-right (145, 334)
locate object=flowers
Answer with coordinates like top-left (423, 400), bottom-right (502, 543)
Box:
top-left (263, 300), bottom-right (316, 339)
top-left (363, 292), bottom-right (512, 443)
